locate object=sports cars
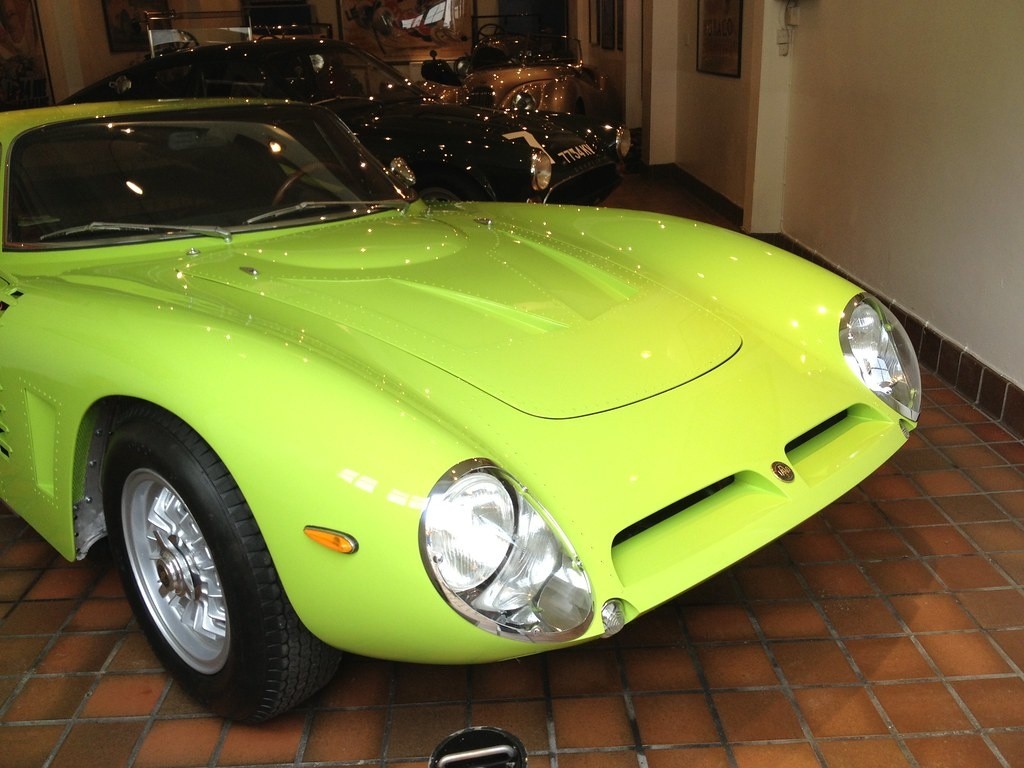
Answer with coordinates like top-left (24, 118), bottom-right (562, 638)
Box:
top-left (58, 39), bottom-right (635, 209)
top-left (0, 98), bottom-right (923, 725)
top-left (419, 30), bottom-right (604, 117)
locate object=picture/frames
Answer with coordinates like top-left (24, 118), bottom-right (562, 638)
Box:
top-left (0, 0), bottom-right (57, 113)
top-left (588, 0), bottom-right (623, 51)
top-left (102, 1), bottom-right (171, 54)
top-left (695, 0), bottom-right (742, 79)
top-left (335, 0), bottom-right (477, 67)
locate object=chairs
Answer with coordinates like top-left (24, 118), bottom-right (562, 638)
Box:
top-left (472, 49), bottom-right (505, 70)
